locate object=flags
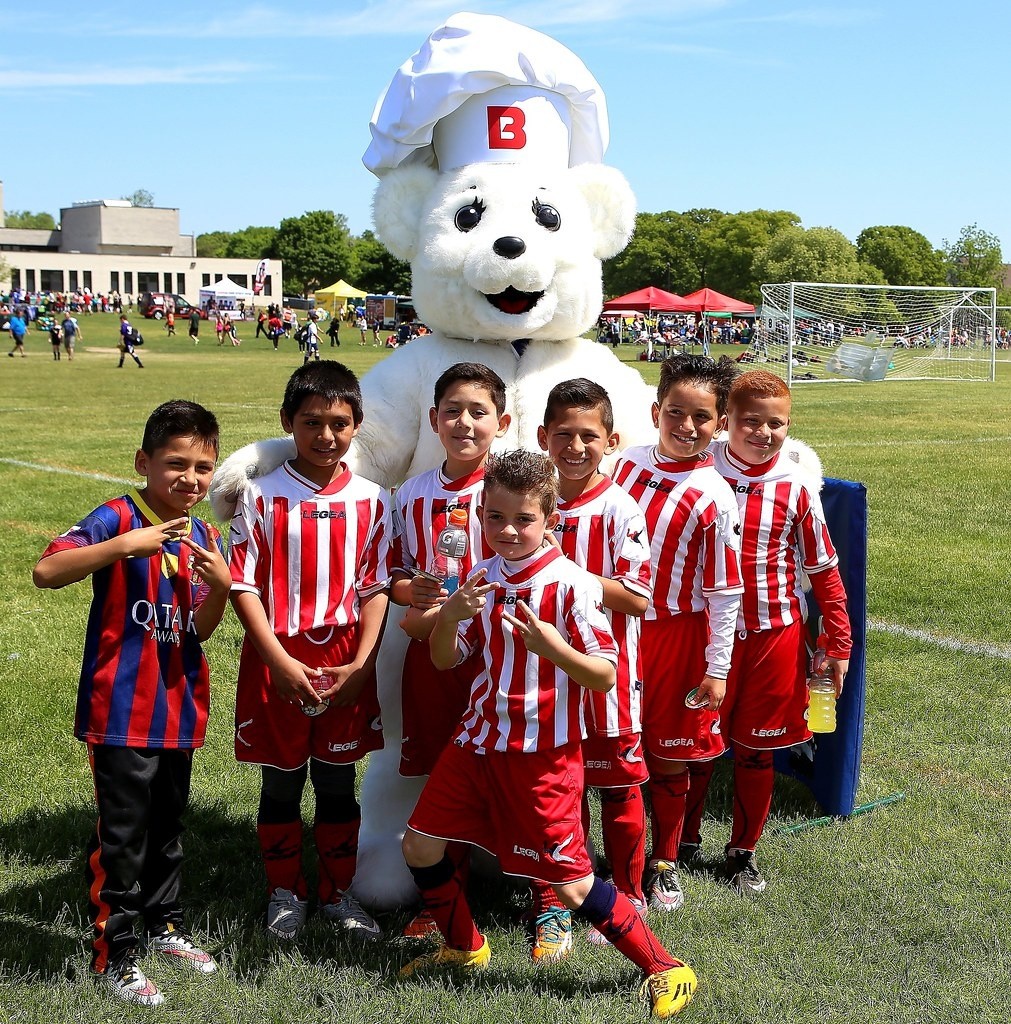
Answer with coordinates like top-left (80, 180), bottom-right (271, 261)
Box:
top-left (254, 258), bottom-right (268, 292)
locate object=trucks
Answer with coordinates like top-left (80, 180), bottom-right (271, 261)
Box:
top-left (366, 294), bottom-right (413, 330)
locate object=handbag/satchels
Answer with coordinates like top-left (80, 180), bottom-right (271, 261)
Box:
top-left (131, 330), bottom-right (143, 345)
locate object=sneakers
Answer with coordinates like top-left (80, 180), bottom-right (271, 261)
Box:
top-left (268, 888), bottom-right (308, 949)
top-left (398, 934), bottom-right (492, 981)
top-left (318, 884), bottom-right (385, 944)
top-left (532, 905), bottom-right (575, 969)
top-left (89, 956), bottom-right (166, 1011)
top-left (647, 859), bottom-right (685, 913)
top-left (725, 848), bottom-right (767, 901)
top-left (403, 905), bottom-right (439, 939)
top-left (519, 906), bottom-right (534, 926)
top-left (676, 842), bottom-right (702, 880)
top-left (638, 957), bottom-right (697, 1019)
top-left (586, 892), bottom-right (647, 947)
top-left (139, 922), bottom-right (218, 976)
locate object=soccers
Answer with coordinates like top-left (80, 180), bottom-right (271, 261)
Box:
top-left (372, 344), bottom-right (377, 348)
top-left (792, 358), bottom-right (799, 368)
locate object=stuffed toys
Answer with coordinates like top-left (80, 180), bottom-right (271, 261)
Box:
top-left (210, 14), bottom-right (824, 911)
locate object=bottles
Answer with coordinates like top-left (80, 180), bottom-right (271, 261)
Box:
top-left (807, 631), bottom-right (836, 733)
top-left (428, 507), bottom-right (469, 606)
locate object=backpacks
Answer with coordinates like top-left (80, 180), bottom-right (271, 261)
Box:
top-left (295, 323), bottom-right (312, 343)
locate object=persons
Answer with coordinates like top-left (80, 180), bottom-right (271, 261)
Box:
top-left (31, 400), bottom-right (230, 1008)
top-left (536, 378), bottom-right (653, 941)
top-left (390, 363), bottom-right (572, 966)
top-left (118, 315), bottom-right (144, 368)
top-left (597, 313), bottom-right (1011, 350)
top-left (229, 359), bottom-right (394, 947)
top-left (601, 354), bottom-right (744, 910)
top-left (401, 453), bottom-right (696, 1016)
top-left (0, 286), bottom-right (427, 364)
top-left (680, 372), bottom-right (852, 895)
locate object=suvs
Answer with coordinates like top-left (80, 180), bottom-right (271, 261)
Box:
top-left (137, 290), bottom-right (205, 321)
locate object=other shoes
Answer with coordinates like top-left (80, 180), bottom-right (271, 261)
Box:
top-left (379, 341), bottom-right (382, 345)
top-left (8, 353), bottom-right (13, 357)
top-left (373, 344), bottom-right (376, 346)
top-left (195, 340), bottom-right (199, 345)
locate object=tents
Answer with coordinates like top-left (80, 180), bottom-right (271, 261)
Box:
top-left (732, 304), bottom-right (789, 321)
top-left (703, 312), bottom-right (732, 324)
top-left (199, 276), bottom-right (254, 309)
top-left (315, 279), bottom-right (367, 320)
top-left (603, 286), bottom-right (705, 361)
top-left (782, 307), bottom-right (821, 320)
top-left (601, 310), bottom-right (647, 344)
top-left (657, 288), bottom-right (758, 349)
